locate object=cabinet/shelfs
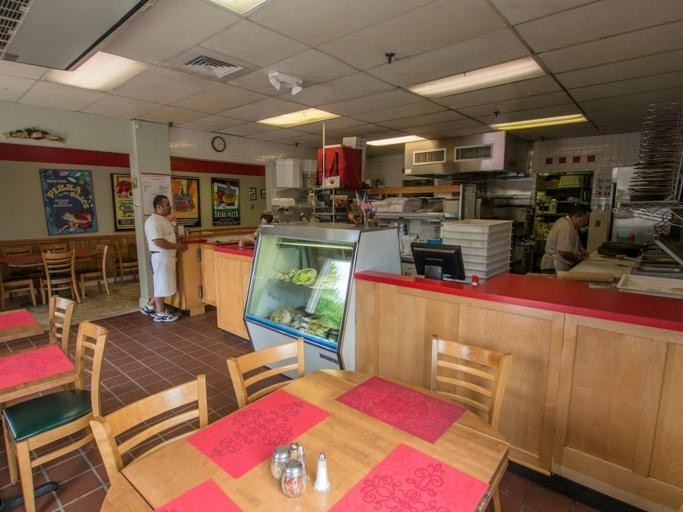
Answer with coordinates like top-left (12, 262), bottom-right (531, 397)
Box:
top-left (243, 221), bottom-right (402, 380)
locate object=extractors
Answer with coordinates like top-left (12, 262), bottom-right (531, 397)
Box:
top-left (403, 129), bottom-right (528, 179)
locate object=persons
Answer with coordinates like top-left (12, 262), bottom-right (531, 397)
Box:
top-left (539, 201), bottom-right (592, 274)
top-left (140, 195), bottom-right (188, 322)
top-left (237, 213), bottom-right (273, 247)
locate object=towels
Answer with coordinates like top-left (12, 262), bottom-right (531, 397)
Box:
top-left (327, 444), bottom-right (489, 512)
top-left (187, 389), bottom-right (331, 479)
top-left (154, 478), bottom-right (243, 512)
top-left (334, 376), bottom-right (466, 444)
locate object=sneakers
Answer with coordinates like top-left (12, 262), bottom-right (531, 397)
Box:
top-left (140, 305), bottom-right (156, 318)
top-left (153, 312), bottom-right (179, 322)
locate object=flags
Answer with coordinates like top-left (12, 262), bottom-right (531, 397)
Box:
top-left (360, 193), bottom-right (370, 213)
top-left (356, 193), bottom-right (363, 206)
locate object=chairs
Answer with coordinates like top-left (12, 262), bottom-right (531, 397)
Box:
top-left (226, 337), bottom-right (304, 409)
top-left (430, 334), bottom-right (513, 431)
top-left (88, 373), bottom-right (208, 485)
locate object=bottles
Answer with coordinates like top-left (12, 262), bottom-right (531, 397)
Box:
top-left (267, 443), bottom-right (308, 501)
top-left (314, 454), bottom-right (330, 492)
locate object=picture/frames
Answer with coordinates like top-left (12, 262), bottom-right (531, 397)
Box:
top-left (110, 173), bottom-right (135, 232)
top-left (171, 175), bottom-right (201, 227)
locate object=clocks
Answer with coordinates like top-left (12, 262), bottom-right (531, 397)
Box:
top-left (212, 136), bottom-right (226, 152)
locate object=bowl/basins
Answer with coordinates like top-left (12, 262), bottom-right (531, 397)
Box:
top-left (267, 307), bottom-right (295, 324)
top-left (306, 317), bottom-right (332, 334)
top-left (292, 268), bottom-right (316, 286)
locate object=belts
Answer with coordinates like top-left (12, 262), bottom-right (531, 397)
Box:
top-left (151, 251), bottom-right (160, 253)
